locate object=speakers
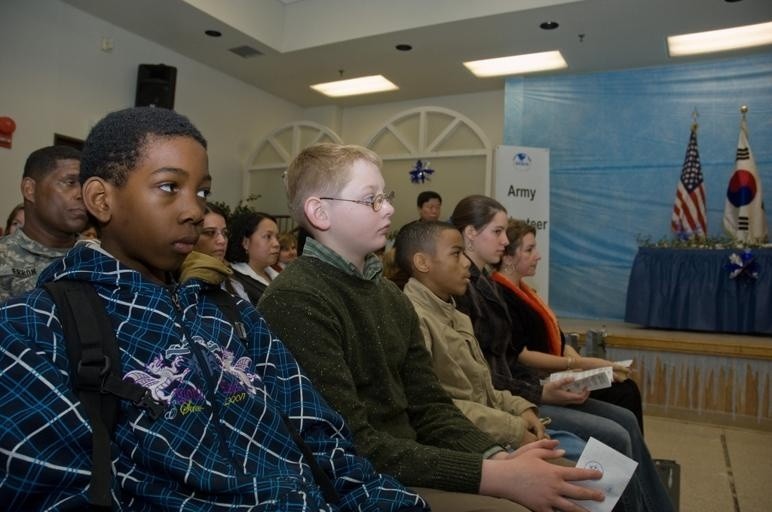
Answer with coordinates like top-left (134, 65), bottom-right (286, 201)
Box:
top-left (135, 63), bottom-right (178, 110)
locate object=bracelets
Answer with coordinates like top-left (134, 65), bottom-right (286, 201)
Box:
top-left (564, 354), bottom-right (577, 370)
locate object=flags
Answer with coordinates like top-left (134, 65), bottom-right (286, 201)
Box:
top-left (672, 127), bottom-right (709, 245)
top-left (723, 124), bottom-right (766, 247)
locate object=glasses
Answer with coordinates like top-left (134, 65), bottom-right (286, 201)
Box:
top-left (311, 190), bottom-right (397, 210)
top-left (202, 228), bottom-right (233, 240)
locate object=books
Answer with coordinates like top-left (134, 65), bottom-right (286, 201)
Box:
top-left (565, 436), bottom-right (640, 512)
top-left (611, 358), bottom-right (635, 367)
top-left (549, 366), bottom-right (614, 394)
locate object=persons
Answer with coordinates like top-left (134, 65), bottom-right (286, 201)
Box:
top-left (191, 200), bottom-right (243, 307)
top-left (398, 191), bottom-right (454, 247)
top-left (492, 218), bottom-right (644, 439)
top-left (392, 218), bottom-right (657, 512)
top-left (0, 106), bottom-right (430, 512)
top-left (223, 211), bottom-right (282, 310)
top-left (446, 193), bottom-right (674, 512)
top-left (255, 142), bottom-right (606, 511)
top-left (5, 203), bottom-right (27, 238)
top-left (273, 232), bottom-right (299, 274)
top-left (0, 146), bottom-right (91, 302)
